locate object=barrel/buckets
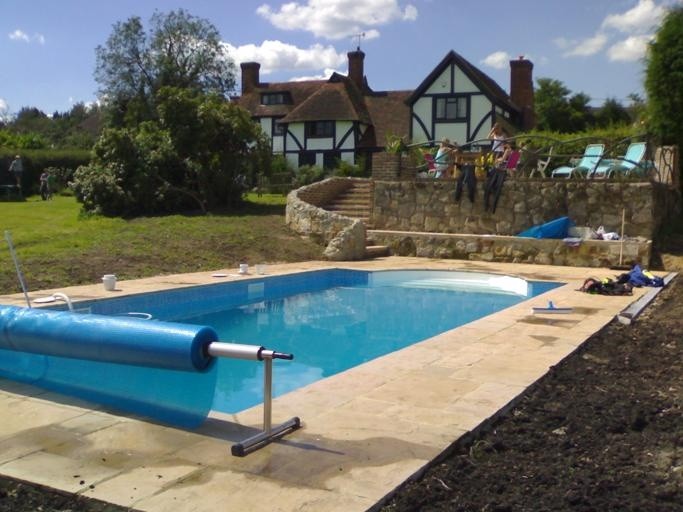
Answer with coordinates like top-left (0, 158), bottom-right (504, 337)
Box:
top-left (101, 274), bottom-right (116, 291)
top-left (239, 264), bottom-right (248, 274)
top-left (254, 264), bottom-right (265, 275)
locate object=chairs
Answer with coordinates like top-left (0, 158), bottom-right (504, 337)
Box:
top-left (425, 142), bottom-right (653, 180)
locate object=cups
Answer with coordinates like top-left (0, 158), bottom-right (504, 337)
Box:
top-left (255, 264), bottom-right (265, 274)
top-left (240, 264), bottom-right (248, 273)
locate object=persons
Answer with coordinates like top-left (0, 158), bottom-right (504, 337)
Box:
top-left (433, 136), bottom-right (457, 171)
top-left (493, 139), bottom-right (516, 166)
top-left (518, 138), bottom-right (543, 160)
top-left (486, 122), bottom-right (508, 158)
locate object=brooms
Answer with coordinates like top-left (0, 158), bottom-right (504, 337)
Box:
top-left (606, 206), bottom-right (633, 270)
